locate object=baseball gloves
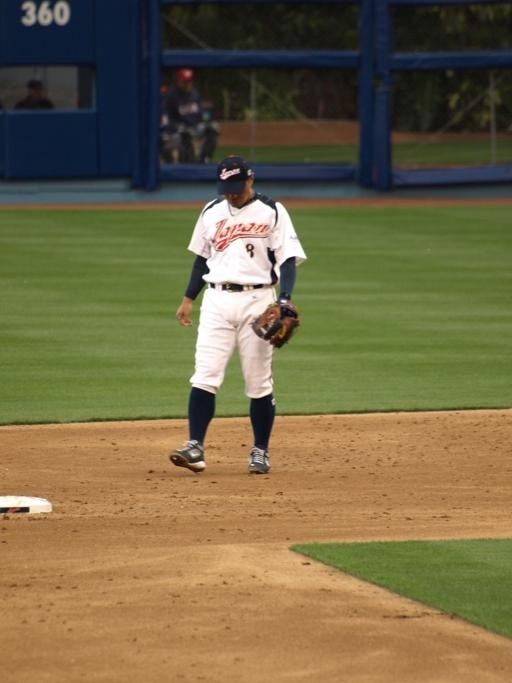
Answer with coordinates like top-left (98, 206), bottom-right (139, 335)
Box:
top-left (251, 299), bottom-right (301, 348)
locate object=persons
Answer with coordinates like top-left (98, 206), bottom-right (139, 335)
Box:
top-left (14, 76), bottom-right (56, 109)
top-left (158, 66), bottom-right (220, 164)
top-left (165, 152), bottom-right (308, 474)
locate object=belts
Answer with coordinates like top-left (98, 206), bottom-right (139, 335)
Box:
top-left (209, 282), bottom-right (263, 291)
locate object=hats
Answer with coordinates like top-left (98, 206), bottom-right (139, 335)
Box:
top-left (215, 154), bottom-right (255, 195)
top-left (176, 69), bottom-right (193, 81)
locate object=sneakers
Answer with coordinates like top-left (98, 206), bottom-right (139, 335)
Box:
top-left (168, 438), bottom-right (207, 473)
top-left (247, 445), bottom-right (272, 473)
